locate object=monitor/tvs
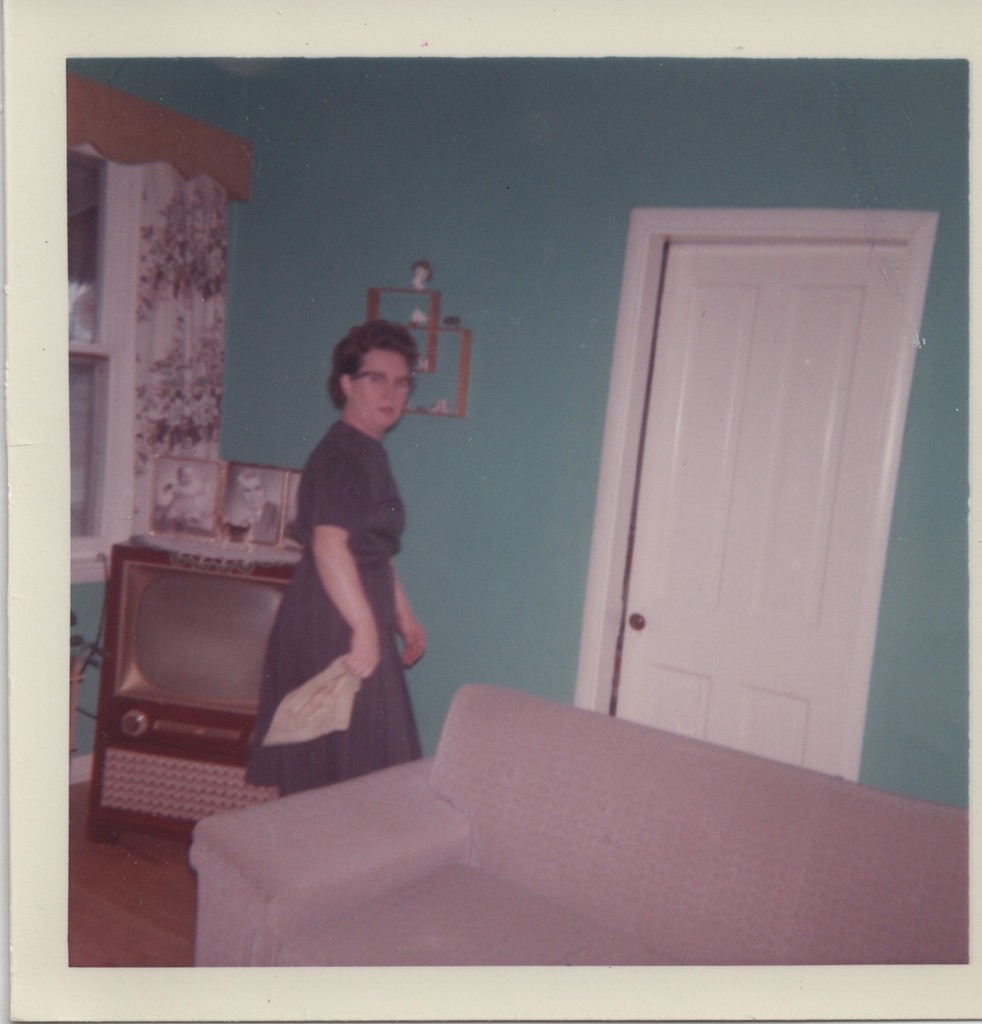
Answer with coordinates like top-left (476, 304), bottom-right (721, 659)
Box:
top-left (97, 535), bottom-right (298, 755)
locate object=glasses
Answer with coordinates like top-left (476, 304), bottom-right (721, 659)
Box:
top-left (349, 372), bottom-right (414, 391)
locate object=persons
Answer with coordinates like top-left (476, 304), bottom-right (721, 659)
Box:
top-left (164, 466), bottom-right (202, 534)
top-left (235, 472), bottom-right (278, 542)
top-left (240, 323), bottom-right (424, 792)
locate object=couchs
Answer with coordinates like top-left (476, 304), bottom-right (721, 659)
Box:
top-left (190, 686), bottom-right (970, 966)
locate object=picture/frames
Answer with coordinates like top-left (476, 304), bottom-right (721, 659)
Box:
top-left (149, 453), bottom-right (300, 550)
